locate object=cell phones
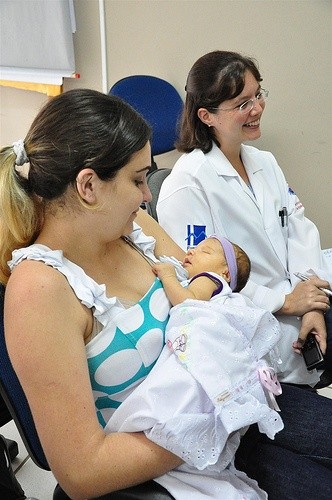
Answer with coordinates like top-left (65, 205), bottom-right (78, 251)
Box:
top-left (300, 333), bottom-right (325, 370)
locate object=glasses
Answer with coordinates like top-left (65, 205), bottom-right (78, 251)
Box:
top-left (207, 88), bottom-right (269, 114)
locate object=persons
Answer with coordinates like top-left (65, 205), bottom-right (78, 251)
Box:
top-left (0, 89), bottom-right (332, 500)
top-left (103, 234), bottom-right (285, 472)
top-left (155, 51), bottom-right (331, 389)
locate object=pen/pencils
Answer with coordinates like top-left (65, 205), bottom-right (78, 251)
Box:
top-left (294, 272), bottom-right (332, 296)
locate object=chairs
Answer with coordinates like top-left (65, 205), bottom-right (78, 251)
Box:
top-left (0, 284), bottom-right (176, 500)
top-left (107, 73), bottom-right (185, 223)
